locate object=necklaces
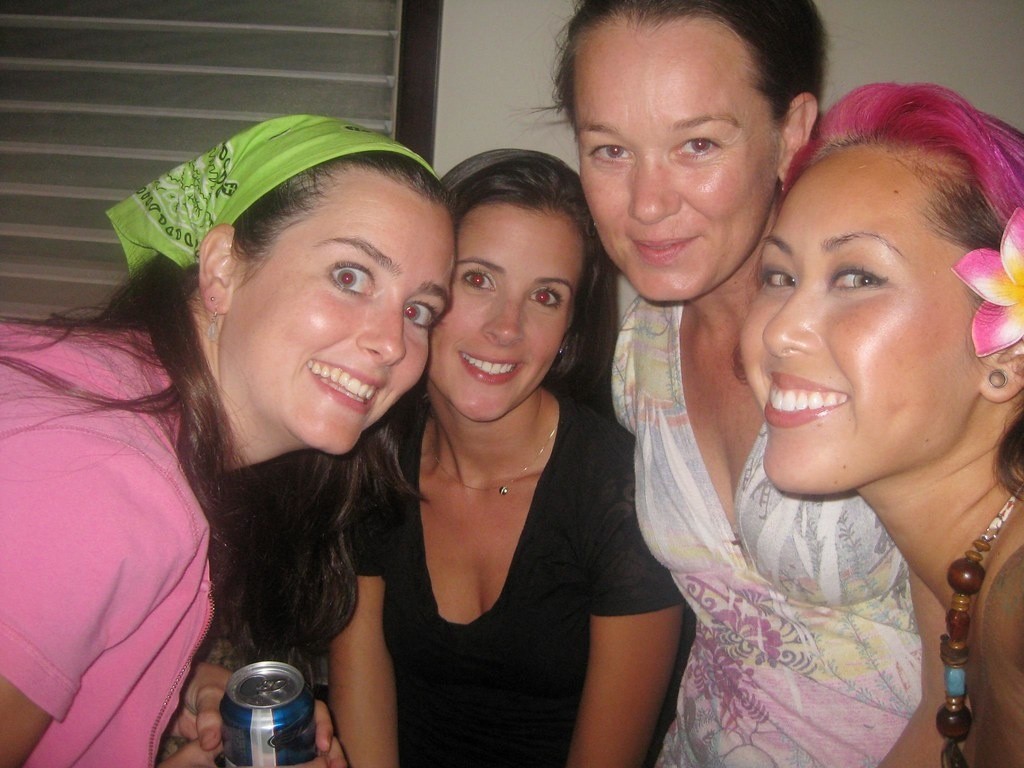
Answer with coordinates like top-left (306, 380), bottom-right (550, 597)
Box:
top-left (936, 486), bottom-right (1024, 768)
top-left (426, 427), bottom-right (558, 495)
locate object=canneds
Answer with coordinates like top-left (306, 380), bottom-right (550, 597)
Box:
top-left (219, 661), bottom-right (319, 768)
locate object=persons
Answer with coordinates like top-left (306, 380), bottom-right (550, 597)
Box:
top-left (0, 113), bottom-right (457, 768)
top-left (739, 82), bottom-right (1024, 768)
top-left (554, 0), bottom-right (924, 768)
top-left (326, 148), bottom-right (688, 768)
